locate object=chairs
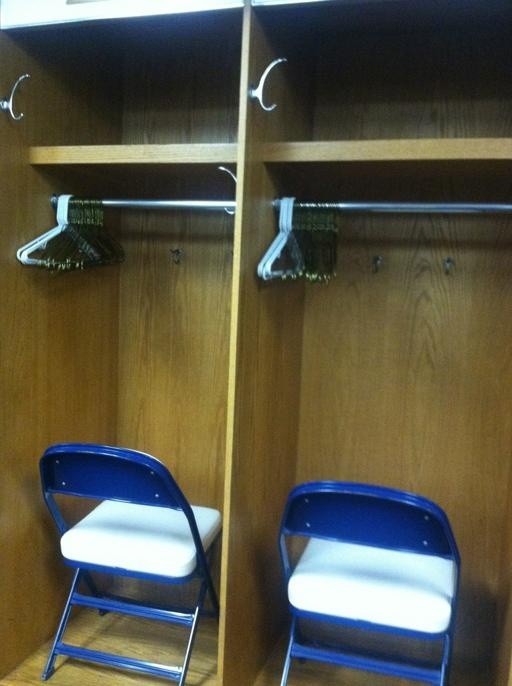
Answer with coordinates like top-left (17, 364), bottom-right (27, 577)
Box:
top-left (37, 441), bottom-right (222, 686)
top-left (278, 479), bottom-right (462, 686)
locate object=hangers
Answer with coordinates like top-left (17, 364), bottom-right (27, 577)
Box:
top-left (254, 197), bottom-right (338, 286)
top-left (14, 194), bottom-right (127, 274)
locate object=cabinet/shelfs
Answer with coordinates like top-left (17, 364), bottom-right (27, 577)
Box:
top-left (0, 0), bottom-right (512, 686)
